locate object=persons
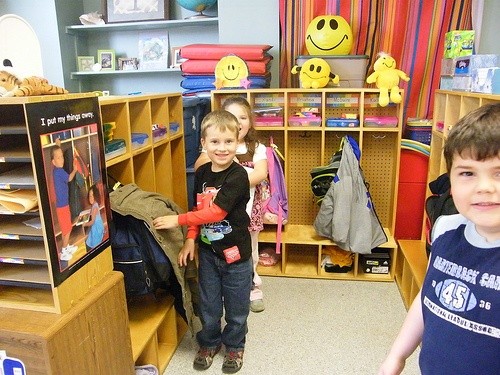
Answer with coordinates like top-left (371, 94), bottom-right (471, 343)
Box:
top-left (50, 138), bottom-right (79, 261)
top-left (82, 185), bottom-right (104, 251)
top-left (194, 98), bottom-right (268, 312)
top-left (378, 103), bottom-right (500, 375)
top-left (154, 110), bottom-right (253, 374)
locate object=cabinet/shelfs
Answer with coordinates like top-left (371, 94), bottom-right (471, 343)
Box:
top-left (64, 17), bottom-right (219, 79)
top-left (0, 88), bottom-right (500, 375)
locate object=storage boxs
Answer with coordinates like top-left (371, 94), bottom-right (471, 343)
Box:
top-left (406, 124), bottom-right (432, 146)
top-left (107, 0), bottom-right (170, 23)
top-left (326, 118), bottom-right (359, 127)
top-left (440, 30), bottom-right (500, 95)
top-left (131, 133), bottom-right (150, 150)
top-left (364, 116), bottom-right (398, 128)
top-left (105, 139), bottom-right (126, 161)
top-left (289, 116), bottom-right (322, 127)
top-left (254, 116), bottom-right (283, 127)
top-left (169, 122), bottom-right (180, 136)
top-left (360, 251), bottom-right (391, 274)
top-left (296, 55), bottom-right (370, 88)
top-left (152, 128), bottom-right (167, 143)
top-left (183, 97), bottom-right (211, 167)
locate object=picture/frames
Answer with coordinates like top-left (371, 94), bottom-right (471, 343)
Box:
top-left (78, 56), bottom-right (95, 72)
top-left (97, 49), bottom-right (115, 71)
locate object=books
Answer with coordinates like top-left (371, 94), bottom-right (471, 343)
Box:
top-left (76, 209), bottom-right (90, 226)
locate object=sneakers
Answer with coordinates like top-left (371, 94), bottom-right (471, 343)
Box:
top-left (222, 349), bottom-right (243, 374)
top-left (250, 298), bottom-right (264, 312)
top-left (194, 347), bottom-right (218, 370)
top-left (60, 252), bottom-right (72, 260)
top-left (62, 244), bottom-right (78, 253)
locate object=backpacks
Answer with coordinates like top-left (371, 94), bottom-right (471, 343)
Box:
top-left (112, 211), bottom-right (172, 298)
top-left (310, 137), bottom-right (344, 207)
top-left (260, 136), bottom-right (289, 225)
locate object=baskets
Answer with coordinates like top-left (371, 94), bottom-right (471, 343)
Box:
top-left (407, 121), bottom-right (432, 145)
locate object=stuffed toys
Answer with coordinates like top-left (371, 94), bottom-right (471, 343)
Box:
top-left (290, 58), bottom-right (339, 88)
top-left (0, 71), bottom-right (69, 98)
top-left (367, 52), bottom-right (411, 107)
